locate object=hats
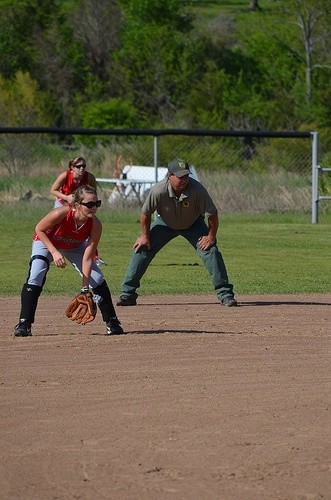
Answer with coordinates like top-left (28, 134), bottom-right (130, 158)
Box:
top-left (168, 159), bottom-right (192, 178)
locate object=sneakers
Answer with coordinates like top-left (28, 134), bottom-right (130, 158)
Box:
top-left (14, 319), bottom-right (31, 336)
top-left (116, 295), bottom-right (136, 306)
top-left (221, 296), bottom-right (237, 307)
top-left (106, 318), bottom-right (123, 335)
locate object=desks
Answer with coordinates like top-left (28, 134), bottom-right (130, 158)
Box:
top-left (95, 178), bottom-right (158, 203)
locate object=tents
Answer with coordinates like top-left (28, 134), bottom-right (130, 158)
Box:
top-left (108, 165), bottom-right (198, 203)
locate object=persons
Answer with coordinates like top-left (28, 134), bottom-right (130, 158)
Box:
top-left (14, 185), bottom-right (123, 336)
top-left (116, 159), bottom-right (237, 307)
top-left (51, 157), bottom-right (107, 266)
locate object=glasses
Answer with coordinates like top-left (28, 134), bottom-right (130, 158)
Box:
top-left (80, 200), bottom-right (101, 208)
top-left (74, 164), bottom-right (86, 168)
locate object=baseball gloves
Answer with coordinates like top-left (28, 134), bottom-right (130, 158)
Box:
top-left (65, 291), bottom-right (98, 325)
